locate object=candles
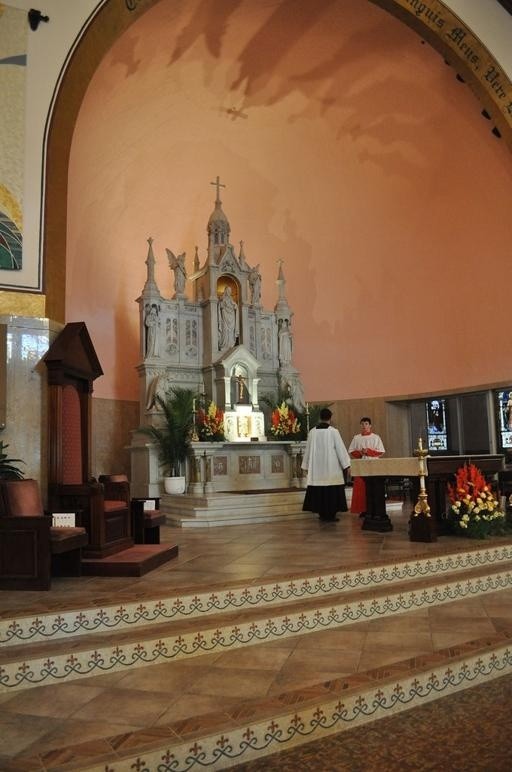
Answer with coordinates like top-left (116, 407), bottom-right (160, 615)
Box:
top-left (418, 436), bottom-right (422, 449)
top-left (306, 402), bottom-right (308, 412)
top-left (193, 397), bottom-right (195, 410)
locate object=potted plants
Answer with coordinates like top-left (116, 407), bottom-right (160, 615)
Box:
top-left (131, 387), bottom-right (195, 495)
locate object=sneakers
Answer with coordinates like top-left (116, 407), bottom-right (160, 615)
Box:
top-left (319, 517), bottom-right (339, 522)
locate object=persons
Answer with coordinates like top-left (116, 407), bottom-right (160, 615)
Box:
top-left (279, 321), bottom-right (294, 366)
top-left (301, 408), bottom-right (351, 522)
top-left (145, 308), bottom-right (162, 357)
top-left (166, 249), bottom-right (190, 295)
top-left (432, 411), bottom-right (441, 430)
top-left (233, 375), bottom-right (246, 399)
top-left (505, 392), bottom-right (511, 432)
top-left (218, 285), bottom-right (239, 350)
top-left (247, 263), bottom-right (262, 306)
top-left (348, 417), bottom-right (386, 517)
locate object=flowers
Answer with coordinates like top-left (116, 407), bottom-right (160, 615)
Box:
top-left (446, 463), bottom-right (505, 539)
top-left (270, 400), bottom-right (302, 440)
top-left (198, 400), bottom-right (225, 441)
